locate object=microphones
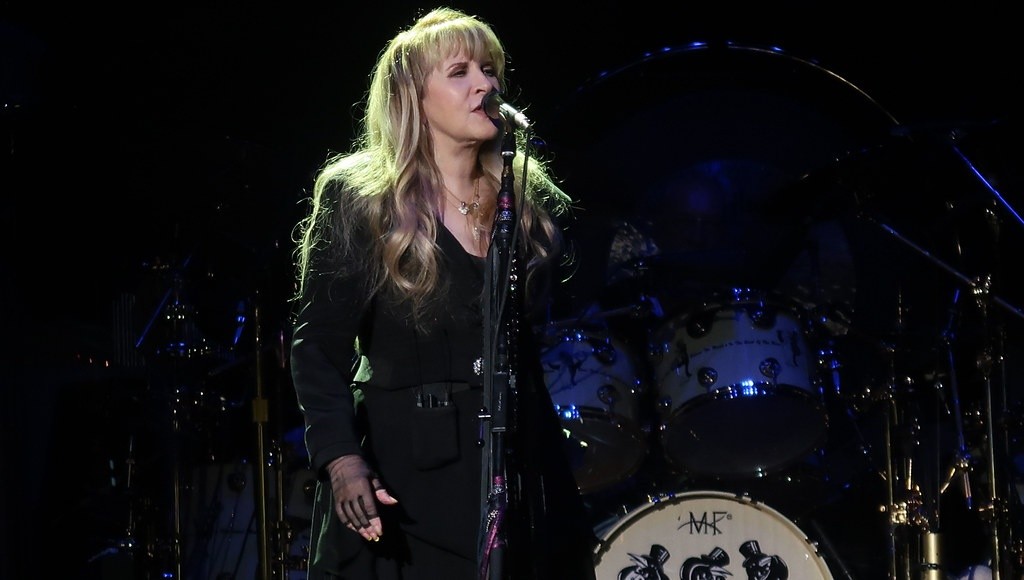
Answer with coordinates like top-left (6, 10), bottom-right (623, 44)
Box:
top-left (482, 92), bottom-right (532, 132)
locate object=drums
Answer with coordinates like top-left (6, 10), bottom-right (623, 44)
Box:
top-left (538, 327), bottom-right (655, 502)
top-left (180, 456), bottom-right (319, 579)
top-left (659, 284), bottom-right (832, 525)
top-left (584, 486), bottom-right (835, 580)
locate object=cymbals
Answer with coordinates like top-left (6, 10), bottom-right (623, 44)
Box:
top-left (577, 213), bottom-right (660, 288)
top-left (797, 117), bottom-right (989, 192)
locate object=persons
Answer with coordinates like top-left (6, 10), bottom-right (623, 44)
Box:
top-left (288, 7), bottom-right (581, 580)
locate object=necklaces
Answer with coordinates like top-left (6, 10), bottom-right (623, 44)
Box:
top-left (442, 170), bottom-right (479, 241)
